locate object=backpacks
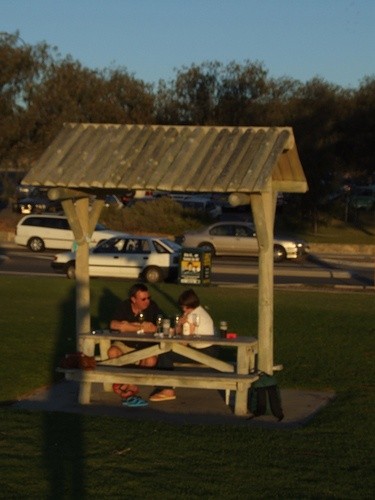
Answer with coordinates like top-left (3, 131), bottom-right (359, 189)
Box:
top-left (248, 372), bottom-right (284, 422)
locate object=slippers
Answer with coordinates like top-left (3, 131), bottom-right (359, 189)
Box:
top-left (113, 384), bottom-right (139, 398)
top-left (122, 396), bottom-right (148, 406)
top-left (149, 394), bottom-right (176, 401)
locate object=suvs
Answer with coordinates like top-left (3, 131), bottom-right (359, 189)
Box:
top-left (15, 213), bottom-right (138, 251)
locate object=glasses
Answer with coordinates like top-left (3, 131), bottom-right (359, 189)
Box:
top-left (142, 296), bottom-right (151, 301)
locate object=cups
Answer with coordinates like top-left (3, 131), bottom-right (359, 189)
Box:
top-left (162, 319), bottom-right (170, 335)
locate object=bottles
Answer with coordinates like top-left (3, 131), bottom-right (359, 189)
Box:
top-left (219, 320), bottom-right (228, 340)
top-left (175, 312), bottom-right (192, 336)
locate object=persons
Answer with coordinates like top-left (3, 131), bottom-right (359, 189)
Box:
top-left (109, 284), bottom-right (169, 397)
top-left (149, 289), bottom-right (215, 401)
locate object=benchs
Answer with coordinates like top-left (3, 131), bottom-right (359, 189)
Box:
top-left (58, 364), bottom-right (259, 415)
top-left (95, 355), bottom-right (284, 407)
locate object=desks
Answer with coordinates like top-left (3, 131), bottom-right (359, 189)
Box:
top-left (79, 330), bottom-right (259, 414)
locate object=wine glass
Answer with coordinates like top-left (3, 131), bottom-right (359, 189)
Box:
top-left (136, 313), bottom-right (146, 336)
top-left (155, 314), bottom-right (163, 337)
top-left (192, 313), bottom-right (202, 338)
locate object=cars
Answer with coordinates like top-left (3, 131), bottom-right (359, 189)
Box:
top-left (178, 221), bottom-right (305, 263)
top-left (50, 234), bottom-right (183, 282)
